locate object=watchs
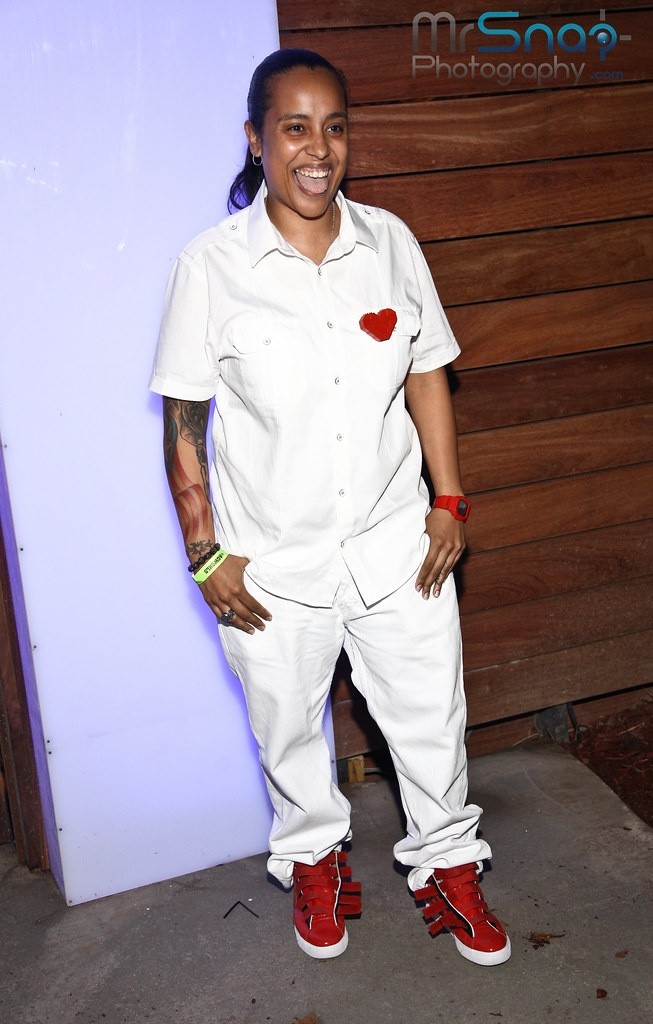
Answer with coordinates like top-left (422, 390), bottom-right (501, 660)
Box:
top-left (433, 495), bottom-right (471, 522)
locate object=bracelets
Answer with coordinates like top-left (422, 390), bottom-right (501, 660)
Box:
top-left (187, 543), bottom-right (220, 572)
top-left (191, 548), bottom-right (228, 584)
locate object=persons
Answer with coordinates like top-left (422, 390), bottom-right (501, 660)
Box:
top-left (150, 49), bottom-right (512, 966)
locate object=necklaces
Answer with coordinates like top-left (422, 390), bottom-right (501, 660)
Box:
top-left (328, 201), bottom-right (336, 249)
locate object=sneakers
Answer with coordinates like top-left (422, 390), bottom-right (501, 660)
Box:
top-left (291, 848), bottom-right (362, 958)
top-left (412, 859), bottom-right (511, 967)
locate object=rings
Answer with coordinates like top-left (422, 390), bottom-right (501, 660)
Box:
top-left (222, 609), bottom-right (235, 623)
